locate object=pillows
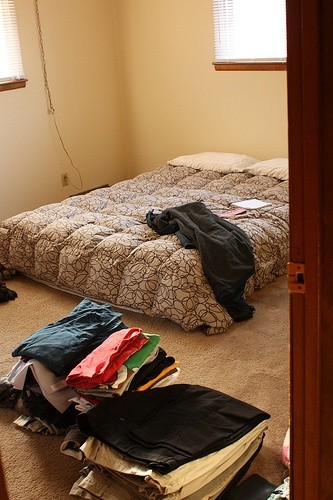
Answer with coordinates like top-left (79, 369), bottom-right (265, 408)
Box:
top-left (167, 152), bottom-right (261, 174)
top-left (242, 158), bottom-right (289, 180)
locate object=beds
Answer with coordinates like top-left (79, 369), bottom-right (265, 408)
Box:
top-left (0, 165), bottom-right (289, 336)
